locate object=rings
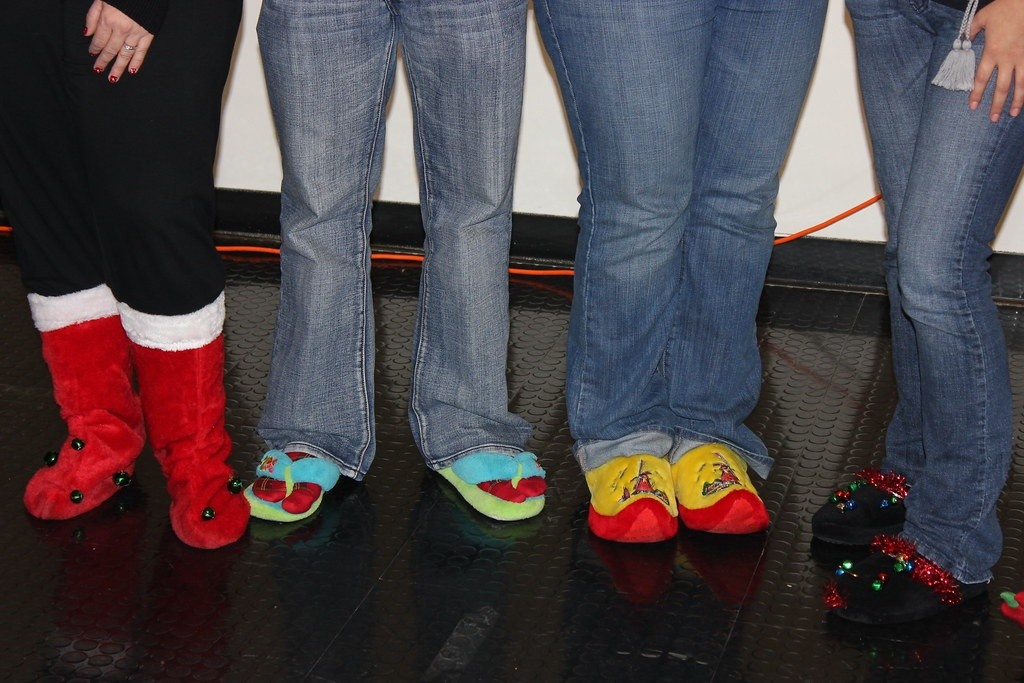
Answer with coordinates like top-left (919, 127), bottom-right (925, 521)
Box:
top-left (123, 41), bottom-right (137, 51)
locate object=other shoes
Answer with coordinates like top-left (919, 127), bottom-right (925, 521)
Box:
top-left (818, 535), bottom-right (984, 624)
top-left (813, 466), bottom-right (913, 544)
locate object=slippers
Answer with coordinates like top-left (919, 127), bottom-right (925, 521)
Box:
top-left (435, 451), bottom-right (544, 521)
top-left (244, 450), bottom-right (340, 522)
top-left (586, 444), bottom-right (769, 542)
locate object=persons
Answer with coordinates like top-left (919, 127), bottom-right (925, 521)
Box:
top-left (241, 0), bottom-right (547, 523)
top-left (811, 0), bottom-right (1024, 623)
top-left (532, 0), bottom-right (830, 543)
top-left (0, 0), bottom-right (251, 550)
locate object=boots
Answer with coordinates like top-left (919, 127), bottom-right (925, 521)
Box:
top-left (24, 284), bottom-right (250, 549)
